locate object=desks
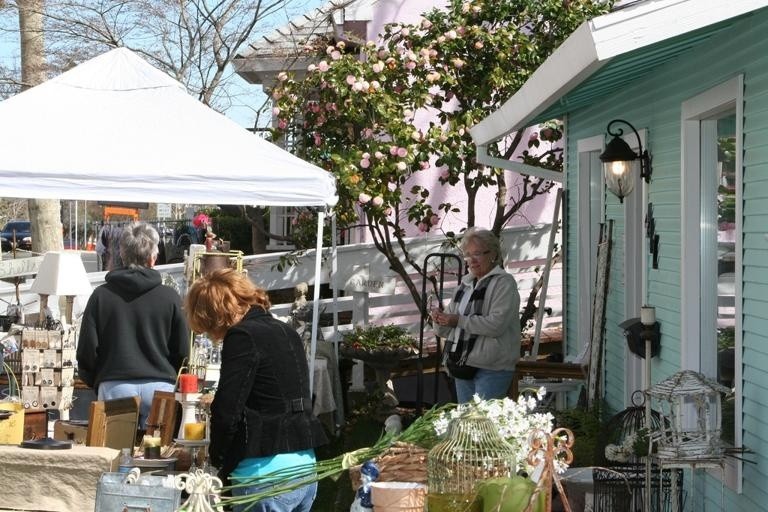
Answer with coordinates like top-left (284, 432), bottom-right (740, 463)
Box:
top-left (0, 444), bottom-right (120, 511)
top-left (512, 362), bottom-right (586, 403)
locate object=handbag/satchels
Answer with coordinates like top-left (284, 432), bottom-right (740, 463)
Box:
top-left (446, 357), bottom-right (477, 381)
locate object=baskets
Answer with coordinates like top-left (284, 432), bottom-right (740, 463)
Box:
top-left (348, 440), bottom-right (506, 492)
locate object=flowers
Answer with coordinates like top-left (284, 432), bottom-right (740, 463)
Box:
top-left (605, 426), bottom-right (759, 468)
top-left (211, 384), bottom-right (568, 512)
top-left (193, 215), bottom-right (212, 231)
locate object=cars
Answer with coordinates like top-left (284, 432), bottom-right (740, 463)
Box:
top-left (155, 224), bottom-right (175, 236)
top-left (63, 239), bottom-right (82, 250)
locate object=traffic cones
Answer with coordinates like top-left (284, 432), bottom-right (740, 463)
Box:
top-left (90, 238), bottom-right (97, 251)
top-left (85, 235), bottom-right (93, 251)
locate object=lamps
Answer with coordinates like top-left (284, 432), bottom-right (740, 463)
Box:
top-left (599, 119), bottom-right (649, 203)
top-left (30, 250), bottom-right (94, 328)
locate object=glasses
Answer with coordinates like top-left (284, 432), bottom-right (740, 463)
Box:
top-left (462, 249), bottom-right (492, 262)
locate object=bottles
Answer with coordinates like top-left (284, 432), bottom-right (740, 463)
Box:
top-left (205, 233), bottom-right (215, 252)
top-left (119, 447), bottom-right (134, 474)
top-left (523, 351), bottom-right (533, 383)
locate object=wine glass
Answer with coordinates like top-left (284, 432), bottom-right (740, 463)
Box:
top-left (189, 447), bottom-right (201, 473)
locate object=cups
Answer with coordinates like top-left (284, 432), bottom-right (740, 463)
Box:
top-left (145, 438), bottom-right (160, 459)
top-left (184, 421), bottom-right (205, 440)
top-left (178, 373), bottom-right (198, 392)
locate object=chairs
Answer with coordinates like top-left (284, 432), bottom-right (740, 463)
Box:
top-left (86, 395), bottom-right (140, 457)
top-left (146, 390), bottom-right (178, 445)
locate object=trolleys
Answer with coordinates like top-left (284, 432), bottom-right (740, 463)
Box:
top-left (403, 252), bottom-right (463, 434)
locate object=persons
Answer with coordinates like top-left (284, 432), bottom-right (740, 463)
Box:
top-left (429, 224), bottom-right (522, 406)
top-left (95, 220), bottom-right (217, 271)
top-left (184, 267), bottom-right (320, 512)
top-left (76, 219), bottom-right (191, 431)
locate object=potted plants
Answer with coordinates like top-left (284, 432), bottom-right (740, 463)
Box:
top-left (718, 327), bottom-right (735, 381)
top-left (718, 137), bottom-right (735, 231)
top-left (341, 323), bottom-right (415, 361)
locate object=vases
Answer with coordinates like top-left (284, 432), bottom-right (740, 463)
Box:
top-left (204, 226), bottom-right (217, 238)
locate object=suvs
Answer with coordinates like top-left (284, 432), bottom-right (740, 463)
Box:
top-left (0, 221), bottom-right (33, 253)
